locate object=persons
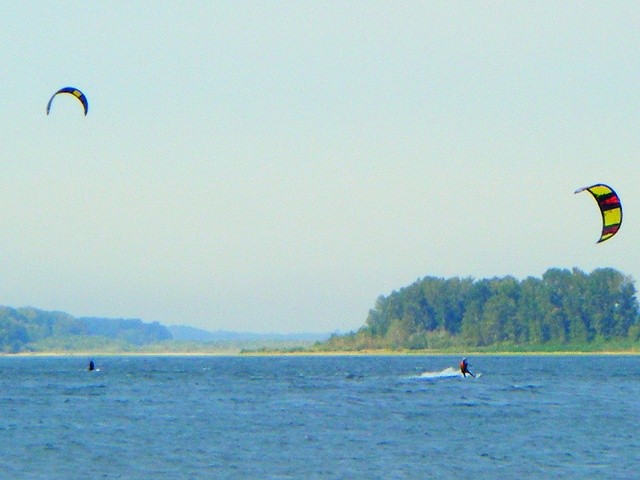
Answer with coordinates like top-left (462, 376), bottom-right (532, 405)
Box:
top-left (460, 358), bottom-right (476, 378)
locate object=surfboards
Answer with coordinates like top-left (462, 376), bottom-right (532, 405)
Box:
top-left (471, 373), bottom-right (481, 378)
